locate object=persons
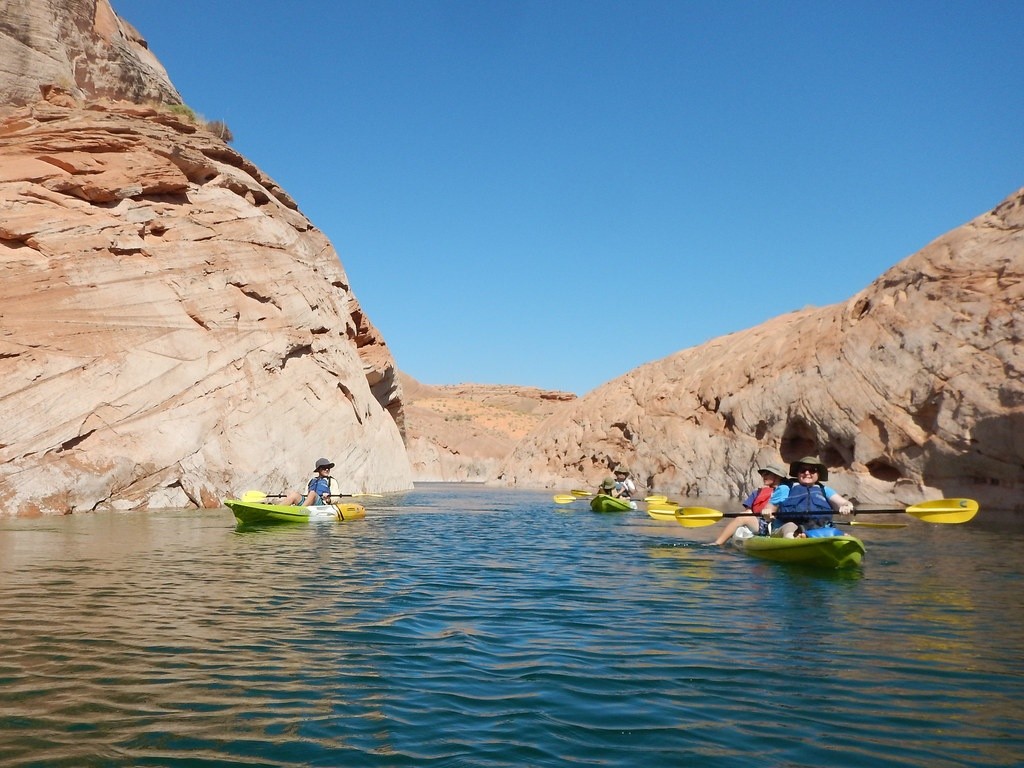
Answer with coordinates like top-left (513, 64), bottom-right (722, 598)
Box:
top-left (715, 455), bottom-right (853, 545)
top-left (280, 457), bottom-right (339, 506)
top-left (600, 467), bottom-right (636, 498)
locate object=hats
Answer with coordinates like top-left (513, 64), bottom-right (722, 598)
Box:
top-left (313, 458), bottom-right (335, 472)
top-left (789, 456), bottom-right (828, 481)
top-left (614, 467), bottom-right (629, 478)
top-left (758, 464), bottom-right (788, 481)
top-left (602, 476), bottom-right (617, 489)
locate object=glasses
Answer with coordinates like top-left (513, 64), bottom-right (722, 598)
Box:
top-left (321, 466), bottom-right (331, 470)
top-left (617, 472), bottom-right (626, 475)
top-left (799, 467), bottom-right (818, 474)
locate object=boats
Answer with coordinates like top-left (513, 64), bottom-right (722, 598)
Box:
top-left (224, 500), bottom-right (369, 524)
top-left (730, 526), bottom-right (871, 569)
top-left (588, 494), bottom-right (635, 513)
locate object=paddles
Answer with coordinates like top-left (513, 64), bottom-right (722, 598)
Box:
top-left (571, 489), bottom-right (680, 506)
top-left (242, 490), bottom-right (383, 504)
top-left (644, 507), bottom-right (910, 531)
top-left (673, 497), bottom-right (980, 530)
top-left (553, 494), bottom-right (667, 506)
